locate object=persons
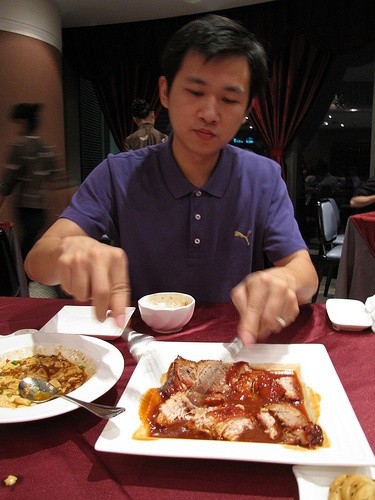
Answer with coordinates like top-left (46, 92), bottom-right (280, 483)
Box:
top-left (24, 13), bottom-right (319, 347)
top-left (348, 176), bottom-right (375, 213)
top-left (0, 103), bottom-right (72, 297)
top-left (122, 98), bottom-right (169, 150)
top-left (300, 157), bottom-right (362, 205)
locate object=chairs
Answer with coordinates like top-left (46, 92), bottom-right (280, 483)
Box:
top-left (312, 197), bottom-right (345, 301)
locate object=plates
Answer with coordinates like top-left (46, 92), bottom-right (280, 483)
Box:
top-left (94, 341), bottom-right (374, 467)
top-left (325, 299), bottom-right (365, 332)
top-left (34, 304), bottom-right (135, 340)
top-left (292, 465), bottom-right (375, 500)
top-left (0, 334), bottom-right (124, 424)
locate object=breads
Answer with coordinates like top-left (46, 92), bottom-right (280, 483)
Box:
top-left (326, 472), bottom-right (375, 500)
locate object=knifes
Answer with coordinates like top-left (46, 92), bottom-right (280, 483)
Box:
top-left (166, 337), bottom-right (245, 423)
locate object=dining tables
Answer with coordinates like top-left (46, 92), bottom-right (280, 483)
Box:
top-left (335, 210), bottom-right (375, 303)
top-left (0, 296), bottom-right (375, 500)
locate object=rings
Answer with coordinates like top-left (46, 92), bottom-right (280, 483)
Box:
top-left (277, 316), bottom-right (288, 328)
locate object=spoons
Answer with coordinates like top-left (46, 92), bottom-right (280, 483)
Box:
top-left (18, 376), bottom-right (126, 420)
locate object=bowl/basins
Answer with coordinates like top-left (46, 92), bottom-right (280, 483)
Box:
top-left (137, 292), bottom-right (196, 335)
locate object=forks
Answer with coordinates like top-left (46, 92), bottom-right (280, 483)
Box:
top-left (104, 308), bottom-right (171, 387)
